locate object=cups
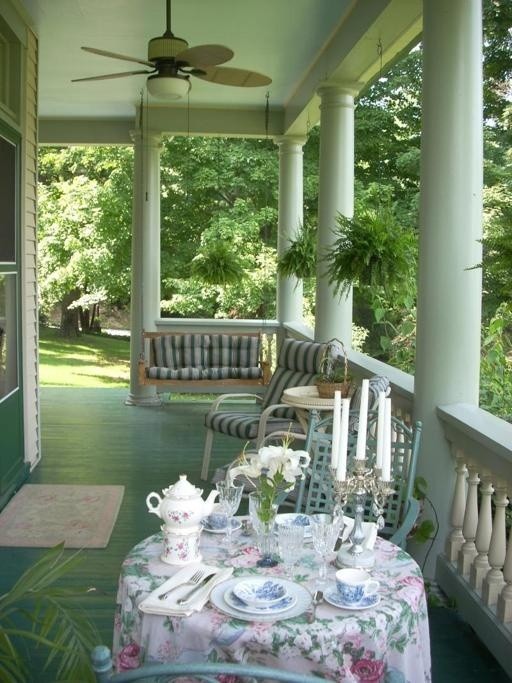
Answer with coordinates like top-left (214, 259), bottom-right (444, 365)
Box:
top-left (334, 564), bottom-right (380, 606)
top-left (207, 502), bottom-right (230, 529)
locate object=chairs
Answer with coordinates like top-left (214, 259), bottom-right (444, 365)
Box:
top-left (200, 338), bottom-right (339, 480)
top-left (294, 406), bottom-right (423, 550)
top-left (256, 374), bottom-right (391, 513)
top-left (91, 644), bottom-right (337, 683)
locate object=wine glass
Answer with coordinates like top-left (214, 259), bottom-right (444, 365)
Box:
top-left (214, 477), bottom-right (344, 584)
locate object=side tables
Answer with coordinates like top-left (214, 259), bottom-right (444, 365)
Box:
top-left (281, 384), bottom-right (356, 436)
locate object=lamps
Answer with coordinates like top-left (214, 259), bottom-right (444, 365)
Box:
top-left (146, 0), bottom-right (193, 98)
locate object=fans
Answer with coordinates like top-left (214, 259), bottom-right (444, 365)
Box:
top-left (70, 1), bottom-right (271, 87)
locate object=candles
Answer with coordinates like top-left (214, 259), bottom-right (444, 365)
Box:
top-left (330, 377), bottom-right (393, 483)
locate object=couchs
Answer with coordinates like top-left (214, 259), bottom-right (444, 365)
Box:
top-left (141, 331), bottom-right (268, 394)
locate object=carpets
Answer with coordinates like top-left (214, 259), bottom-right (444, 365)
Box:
top-left (0, 482), bottom-right (126, 548)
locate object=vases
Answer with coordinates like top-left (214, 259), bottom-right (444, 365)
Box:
top-left (249, 491), bottom-right (281, 556)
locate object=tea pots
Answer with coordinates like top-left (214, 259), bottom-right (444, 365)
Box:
top-left (146, 469), bottom-right (220, 565)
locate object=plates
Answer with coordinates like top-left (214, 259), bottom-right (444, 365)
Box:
top-left (209, 574), bottom-right (313, 623)
top-left (324, 583), bottom-right (382, 611)
top-left (201, 516), bottom-right (242, 534)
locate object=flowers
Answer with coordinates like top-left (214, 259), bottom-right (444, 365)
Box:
top-left (224, 421), bottom-right (311, 524)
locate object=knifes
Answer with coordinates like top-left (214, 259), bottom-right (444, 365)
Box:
top-left (180, 571), bottom-right (217, 604)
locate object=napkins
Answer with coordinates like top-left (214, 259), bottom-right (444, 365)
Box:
top-left (138, 562), bottom-right (231, 619)
top-left (320, 515), bottom-right (378, 558)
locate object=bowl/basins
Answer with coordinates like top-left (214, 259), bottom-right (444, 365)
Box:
top-left (275, 511), bottom-right (314, 532)
top-left (233, 576), bottom-right (290, 607)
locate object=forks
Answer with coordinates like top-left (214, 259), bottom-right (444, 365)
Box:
top-left (159, 567), bottom-right (205, 599)
